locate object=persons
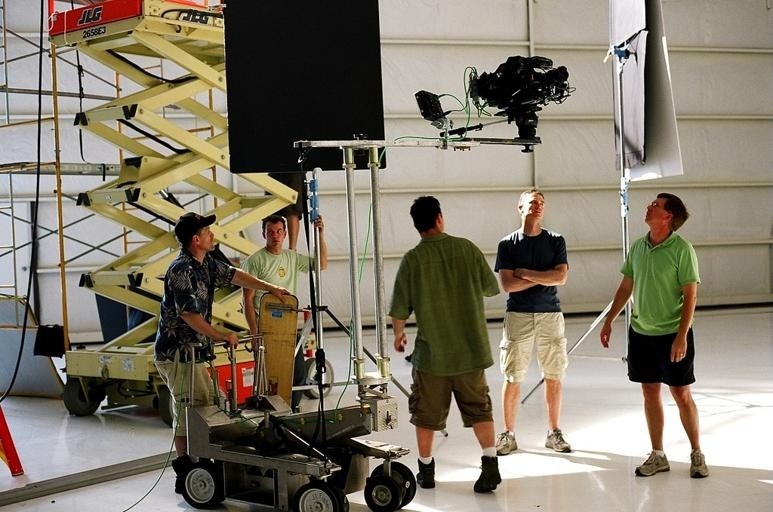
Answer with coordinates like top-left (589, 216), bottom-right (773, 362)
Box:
top-left (388, 196), bottom-right (502, 492)
top-left (154, 211), bottom-right (290, 454)
top-left (493, 189), bottom-right (571, 453)
top-left (243, 214), bottom-right (326, 396)
top-left (599, 193), bottom-right (709, 478)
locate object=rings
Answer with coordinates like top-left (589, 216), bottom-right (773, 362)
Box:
top-left (680, 353), bottom-right (683, 356)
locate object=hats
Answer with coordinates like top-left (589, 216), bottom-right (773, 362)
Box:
top-left (174, 209), bottom-right (216, 243)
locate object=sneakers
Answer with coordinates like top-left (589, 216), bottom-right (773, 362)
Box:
top-left (494, 429), bottom-right (519, 456)
top-left (412, 456), bottom-right (437, 490)
top-left (470, 456), bottom-right (503, 493)
top-left (688, 449), bottom-right (711, 480)
top-left (635, 451), bottom-right (672, 479)
top-left (544, 427), bottom-right (573, 454)
